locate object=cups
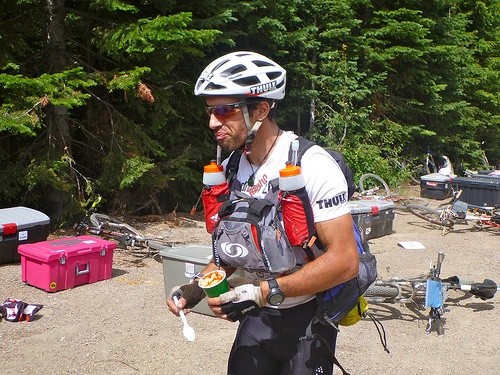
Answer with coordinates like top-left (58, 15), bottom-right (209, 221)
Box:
top-left (198, 270), bottom-right (230, 299)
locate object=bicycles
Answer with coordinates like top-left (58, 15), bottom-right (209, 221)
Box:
top-left (356, 251), bottom-right (500, 333)
top-left (409, 144), bottom-right (438, 185)
top-left (72, 195), bottom-right (173, 258)
top-left (351, 173), bottom-right (500, 237)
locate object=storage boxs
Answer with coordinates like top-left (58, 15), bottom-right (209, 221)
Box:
top-left (349, 199), bottom-right (395, 241)
top-left (450, 176), bottom-right (500, 209)
top-left (420, 173), bottom-right (457, 200)
top-left (0, 206), bottom-right (50, 266)
top-left (17, 236), bottom-right (117, 294)
top-left (159, 244), bottom-right (220, 318)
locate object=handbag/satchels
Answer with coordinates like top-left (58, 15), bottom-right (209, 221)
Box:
top-left (314, 251), bottom-right (376, 333)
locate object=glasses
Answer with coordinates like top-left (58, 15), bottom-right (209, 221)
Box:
top-left (204, 101), bottom-right (253, 118)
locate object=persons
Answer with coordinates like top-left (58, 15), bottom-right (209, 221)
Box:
top-left (166, 52), bottom-right (358, 375)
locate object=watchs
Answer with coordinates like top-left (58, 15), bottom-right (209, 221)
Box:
top-left (266, 278), bottom-right (285, 306)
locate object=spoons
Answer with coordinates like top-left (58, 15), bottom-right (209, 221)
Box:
top-left (173, 295), bottom-right (195, 343)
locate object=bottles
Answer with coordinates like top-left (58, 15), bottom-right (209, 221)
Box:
top-left (279, 160), bottom-right (306, 192)
top-left (202, 160), bottom-right (227, 186)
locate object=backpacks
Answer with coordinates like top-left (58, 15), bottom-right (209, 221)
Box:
top-left (223, 137), bottom-right (379, 325)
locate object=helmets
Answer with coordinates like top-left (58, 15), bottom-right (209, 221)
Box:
top-left (194, 51), bottom-right (287, 100)
